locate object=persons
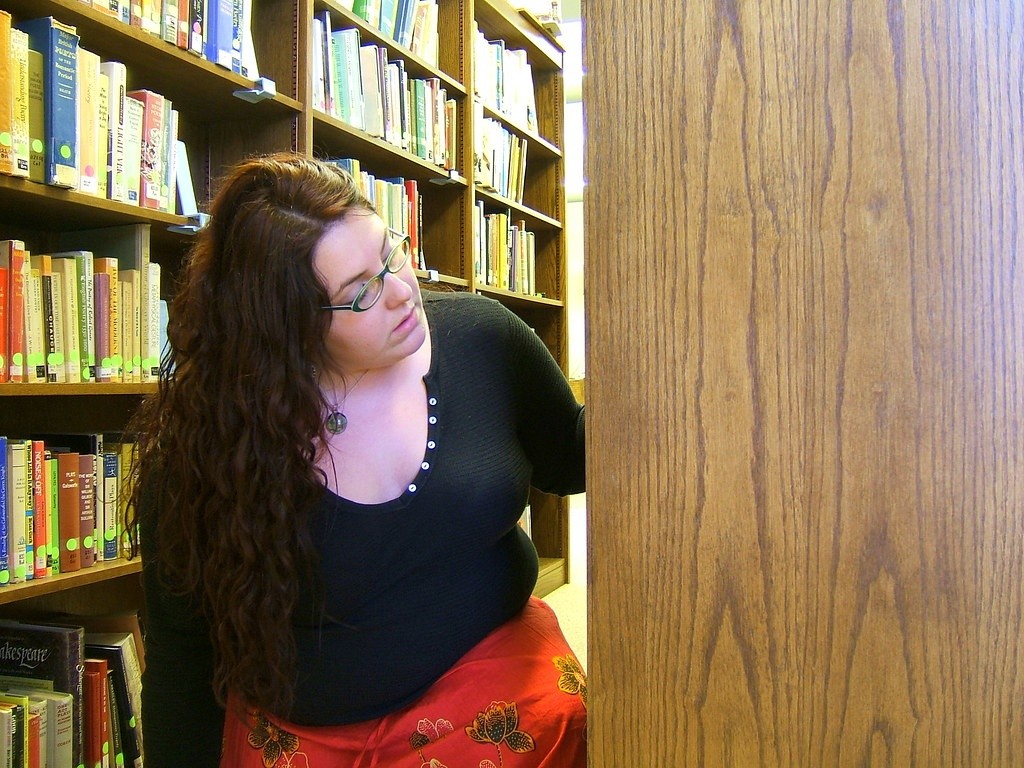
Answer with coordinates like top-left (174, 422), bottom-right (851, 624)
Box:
top-left (142, 156), bottom-right (587, 768)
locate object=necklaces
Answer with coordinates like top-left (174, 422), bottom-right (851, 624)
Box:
top-left (320, 368), bottom-right (369, 436)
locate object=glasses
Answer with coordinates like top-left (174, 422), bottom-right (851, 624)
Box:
top-left (318, 228), bottom-right (411, 312)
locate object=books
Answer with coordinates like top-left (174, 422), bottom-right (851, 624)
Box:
top-left (0, 0), bottom-right (258, 383)
top-left (313, 0), bottom-right (539, 297)
top-left (0, 430), bottom-right (146, 768)
top-left (516, 504), bottom-right (532, 541)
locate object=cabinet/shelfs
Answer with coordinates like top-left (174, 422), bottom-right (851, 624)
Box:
top-left (0, 0), bottom-right (587, 768)
